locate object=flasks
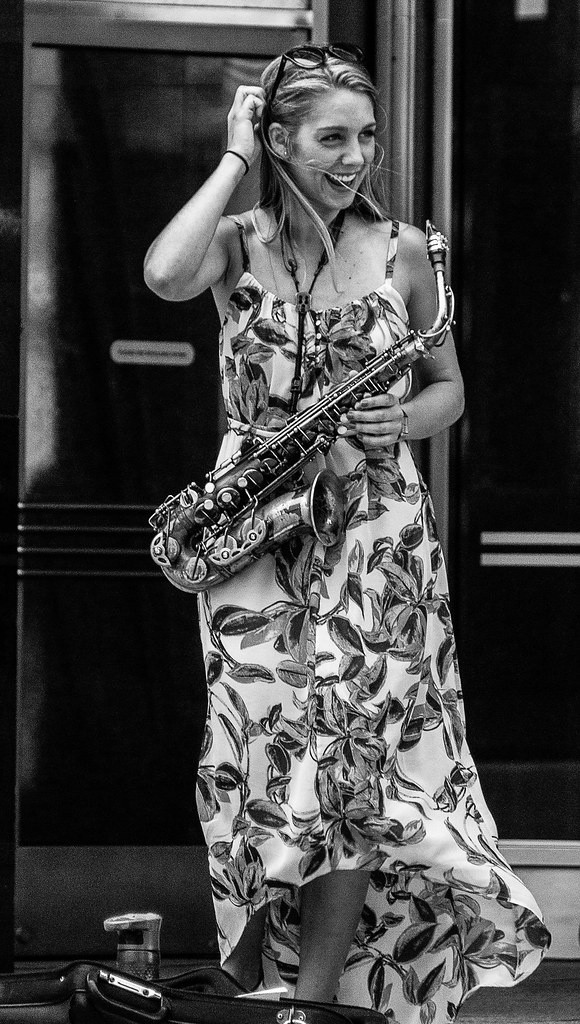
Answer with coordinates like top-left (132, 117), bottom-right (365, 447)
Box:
top-left (103, 911), bottom-right (162, 982)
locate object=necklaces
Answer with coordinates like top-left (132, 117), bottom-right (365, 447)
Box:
top-left (282, 209), bottom-right (345, 413)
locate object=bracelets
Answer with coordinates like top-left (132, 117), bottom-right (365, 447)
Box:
top-left (399, 407), bottom-right (412, 437)
top-left (222, 149), bottom-right (250, 177)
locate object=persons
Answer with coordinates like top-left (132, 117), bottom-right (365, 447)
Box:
top-left (140, 38), bottom-right (554, 1024)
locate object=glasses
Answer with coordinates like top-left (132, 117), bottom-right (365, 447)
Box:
top-left (268, 44), bottom-right (365, 114)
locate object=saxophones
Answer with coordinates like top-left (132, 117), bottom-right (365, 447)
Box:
top-left (146, 218), bottom-right (458, 596)
